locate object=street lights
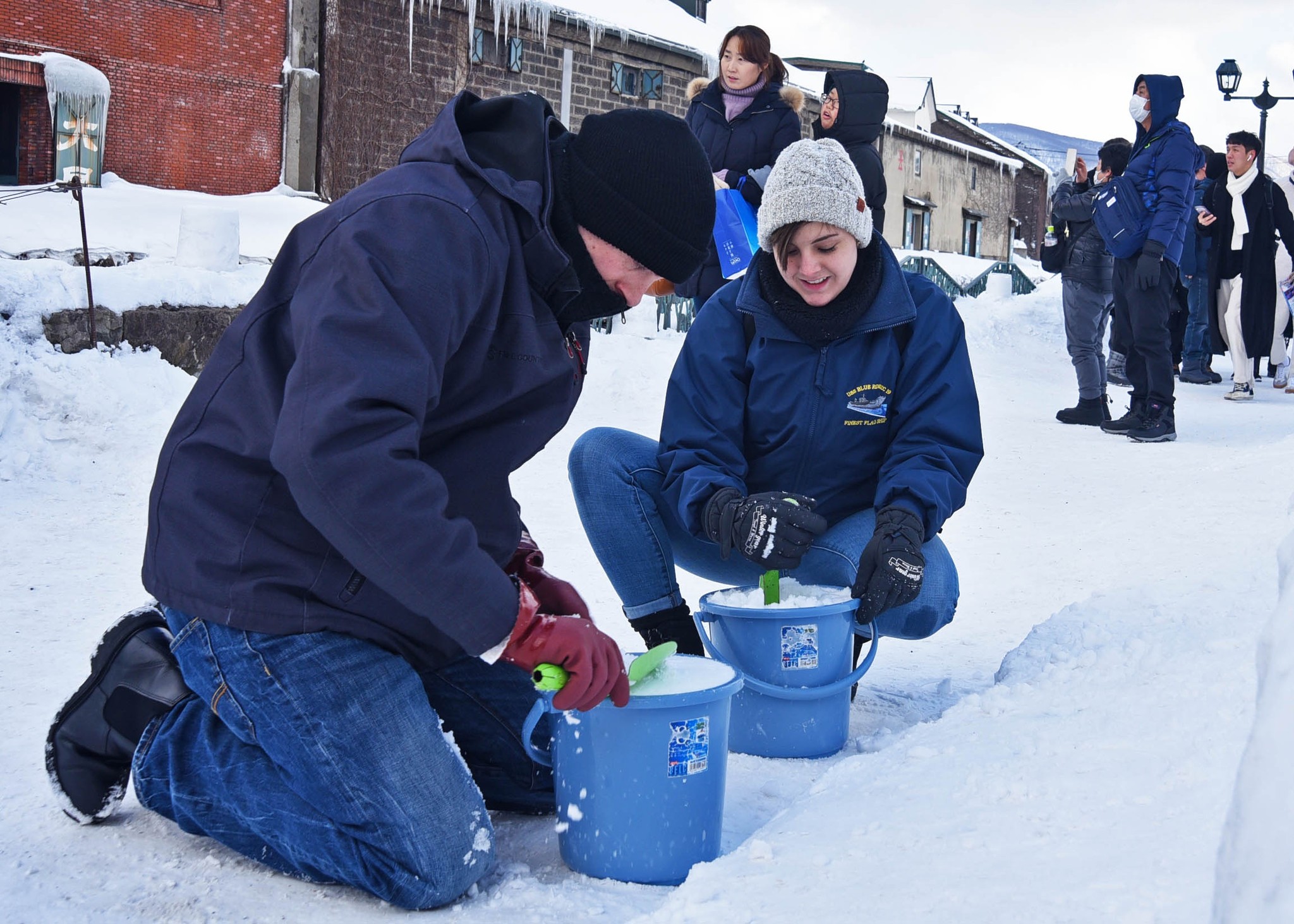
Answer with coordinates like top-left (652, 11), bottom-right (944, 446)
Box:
top-left (1216, 58), bottom-right (1294, 174)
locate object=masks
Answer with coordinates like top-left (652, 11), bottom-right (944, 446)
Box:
top-left (1129, 95), bottom-right (1151, 123)
top-left (1094, 171), bottom-right (1106, 184)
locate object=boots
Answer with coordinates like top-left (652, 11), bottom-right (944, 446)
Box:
top-left (1178, 352), bottom-right (1222, 384)
top-left (1057, 394), bottom-right (1112, 425)
top-left (1107, 351), bottom-right (1130, 386)
top-left (638, 614), bottom-right (704, 656)
top-left (43, 606), bottom-right (194, 824)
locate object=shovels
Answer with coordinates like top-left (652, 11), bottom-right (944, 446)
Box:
top-left (529, 641), bottom-right (680, 696)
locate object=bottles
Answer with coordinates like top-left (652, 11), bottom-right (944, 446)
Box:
top-left (1044, 226), bottom-right (1058, 246)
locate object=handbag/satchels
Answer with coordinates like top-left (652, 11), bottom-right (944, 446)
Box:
top-left (1038, 232), bottom-right (1067, 272)
top-left (712, 177), bottom-right (761, 280)
top-left (1091, 175), bottom-right (1150, 259)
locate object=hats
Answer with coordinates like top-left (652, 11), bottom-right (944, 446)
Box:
top-left (755, 137), bottom-right (872, 252)
top-left (549, 107), bottom-right (716, 284)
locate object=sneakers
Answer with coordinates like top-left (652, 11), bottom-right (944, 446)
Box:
top-left (1127, 400), bottom-right (1177, 442)
top-left (1225, 383), bottom-right (1254, 399)
top-left (1101, 392), bottom-right (1150, 434)
top-left (1273, 355), bottom-right (1294, 393)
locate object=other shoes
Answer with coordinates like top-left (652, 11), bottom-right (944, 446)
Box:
top-left (1230, 373), bottom-right (1234, 380)
top-left (1173, 364), bottom-right (1180, 376)
top-left (1253, 375), bottom-right (1263, 383)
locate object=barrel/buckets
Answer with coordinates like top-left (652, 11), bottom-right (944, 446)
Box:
top-left (693, 584), bottom-right (879, 759)
top-left (521, 652), bottom-right (745, 886)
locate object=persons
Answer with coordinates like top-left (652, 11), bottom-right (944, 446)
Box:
top-left (686, 25), bottom-right (804, 316)
top-left (1100, 74), bottom-right (1205, 443)
top-left (44, 90), bottom-right (717, 910)
top-left (748, 68), bottom-right (888, 236)
top-left (569, 136), bottom-right (984, 701)
top-left (1052, 137), bottom-right (1134, 427)
top-left (1171, 131), bottom-right (1293, 402)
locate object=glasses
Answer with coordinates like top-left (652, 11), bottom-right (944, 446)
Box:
top-left (820, 93), bottom-right (839, 109)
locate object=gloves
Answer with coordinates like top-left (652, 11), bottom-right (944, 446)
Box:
top-left (702, 485), bottom-right (828, 568)
top-left (512, 530), bottom-right (592, 620)
top-left (497, 574), bottom-right (629, 712)
top-left (1133, 240), bottom-right (1166, 290)
top-left (849, 509), bottom-right (926, 625)
